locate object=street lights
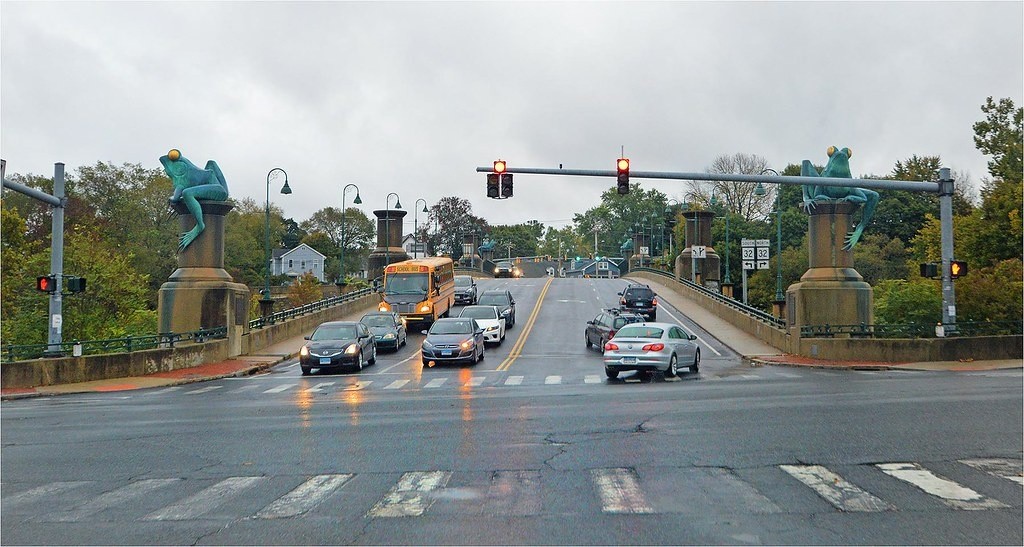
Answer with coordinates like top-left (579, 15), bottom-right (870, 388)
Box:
top-left (414, 198), bottom-right (429, 259)
top-left (680, 193), bottom-right (699, 272)
top-left (754, 168), bottom-right (786, 301)
top-left (262, 168), bottom-right (293, 299)
top-left (386, 193), bottom-right (403, 266)
top-left (710, 185), bottom-right (731, 283)
top-left (664, 198), bottom-right (679, 255)
top-left (618, 203), bottom-right (665, 271)
top-left (337, 183), bottom-right (362, 283)
top-left (434, 209), bottom-right (484, 273)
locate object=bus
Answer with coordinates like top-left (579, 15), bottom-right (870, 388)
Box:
top-left (374, 257), bottom-right (455, 334)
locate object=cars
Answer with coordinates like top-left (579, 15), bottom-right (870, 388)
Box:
top-left (299, 320), bottom-right (378, 375)
top-left (494, 261), bottom-right (515, 278)
top-left (420, 317), bottom-right (486, 367)
top-left (603, 320), bottom-right (701, 381)
top-left (477, 290), bottom-right (515, 329)
top-left (453, 274), bottom-right (478, 305)
top-left (356, 310), bottom-right (407, 352)
top-left (456, 305), bottom-right (507, 347)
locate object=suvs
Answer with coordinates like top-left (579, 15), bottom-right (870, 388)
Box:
top-left (584, 309), bottom-right (647, 356)
top-left (616, 283), bottom-right (657, 321)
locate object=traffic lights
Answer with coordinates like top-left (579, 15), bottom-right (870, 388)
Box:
top-left (68, 277), bottom-right (86, 293)
top-left (501, 173), bottom-right (513, 197)
top-left (617, 159), bottom-right (629, 194)
top-left (486, 173), bottom-right (500, 197)
top-left (920, 263), bottom-right (937, 277)
top-left (950, 260), bottom-right (968, 276)
top-left (36, 275), bottom-right (55, 292)
top-left (494, 161), bottom-right (506, 172)
